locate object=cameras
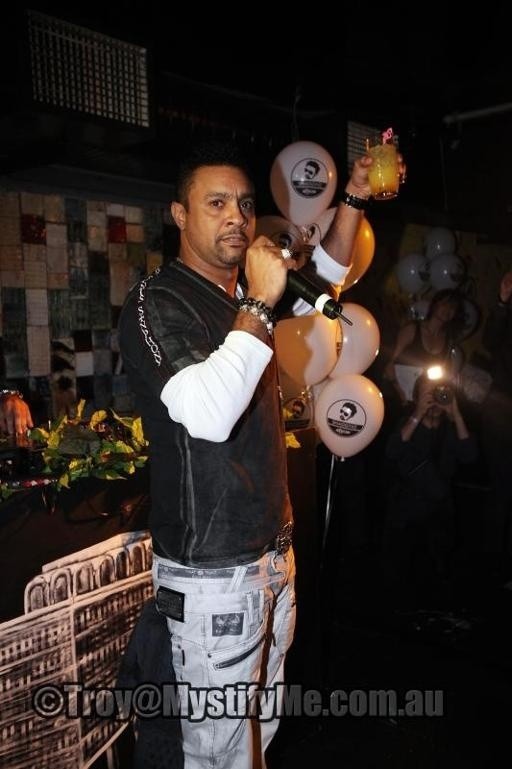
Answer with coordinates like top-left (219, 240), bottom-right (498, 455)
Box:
top-left (434, 385), bottom-right (454, 402)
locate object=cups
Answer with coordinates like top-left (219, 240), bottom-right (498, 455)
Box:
top-left (365, 135), bottom-right (401, 202)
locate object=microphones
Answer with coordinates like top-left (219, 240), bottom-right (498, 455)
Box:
top-left (286, 268), bottom-right (353, 327)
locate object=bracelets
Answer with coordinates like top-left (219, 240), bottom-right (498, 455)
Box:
top-left (340, 190), bottom-right (368, 211)
top-left (238, 296), bottom-right (281, 340)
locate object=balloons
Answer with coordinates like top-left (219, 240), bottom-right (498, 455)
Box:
top-left (274, 312), bottom-right (344, 387)
top-left (316, 373), bottom-right (386, 461)
top-left (268, 139), bottom-right (337, 229)
top-left (238, 216), bottom-right (302, 272)
top-left (326, 301), bottom-right (381, 378)
top-left (278, 376), bottom-right (330, 430)
top-left (304, 204), bottom-right (377, 291)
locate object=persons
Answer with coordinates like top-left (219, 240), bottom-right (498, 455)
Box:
top-left (115, 157), bottom-right (315, 769)
top-left (0, 332), bottom-right (54, 490)
top-left (386, 288), bottom-right (472, 408)
top-left (480, 267), bottom-right (512, 403)
top-left (376, 375), bottom-right (479, 626)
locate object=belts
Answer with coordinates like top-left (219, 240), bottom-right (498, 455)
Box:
top-left (151, 524), bottom-right (293, 564)
